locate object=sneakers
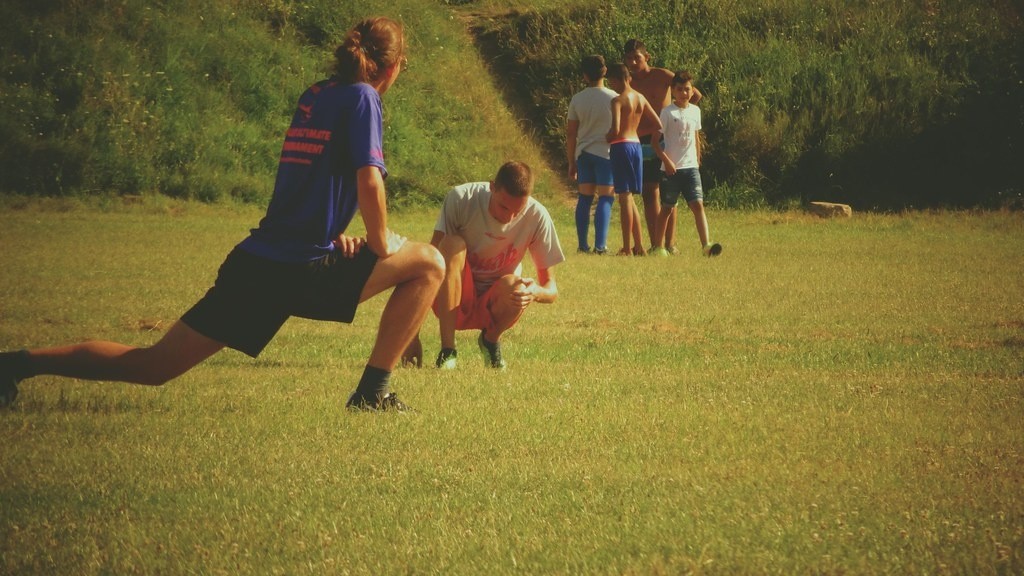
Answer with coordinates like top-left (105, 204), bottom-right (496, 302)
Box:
top-left (478, 328), bottom-right (503, 369)
top-left (577, 245), bottom-right (610, 256)
top-left (616, 247), bottom-right (647, 257)
top-left (345, 391), bottom-right (422, 416)
top-left (436, 348), bottom-right (458, 370)
top-left (0, 352), bottom-right (18, 408)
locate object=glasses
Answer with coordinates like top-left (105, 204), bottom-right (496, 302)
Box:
top-left (400, 55), bottom-right (408, 72)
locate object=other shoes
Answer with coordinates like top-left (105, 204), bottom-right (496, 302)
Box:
top-left (666, 247), bottom-right (682, 256)
top-left (650, 249), bottom-right (669, 257)
top-left (646, 246), bottom-right (655, 254)
top-left (702, 243), bottom-right (723, 258)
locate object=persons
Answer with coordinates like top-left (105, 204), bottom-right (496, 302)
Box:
top-left (0, 17), bottom-right (446, 414)
top-left (401, 160), bottom-right (567, 370)
top-left (566, 39), bottom-right (723, 258)
